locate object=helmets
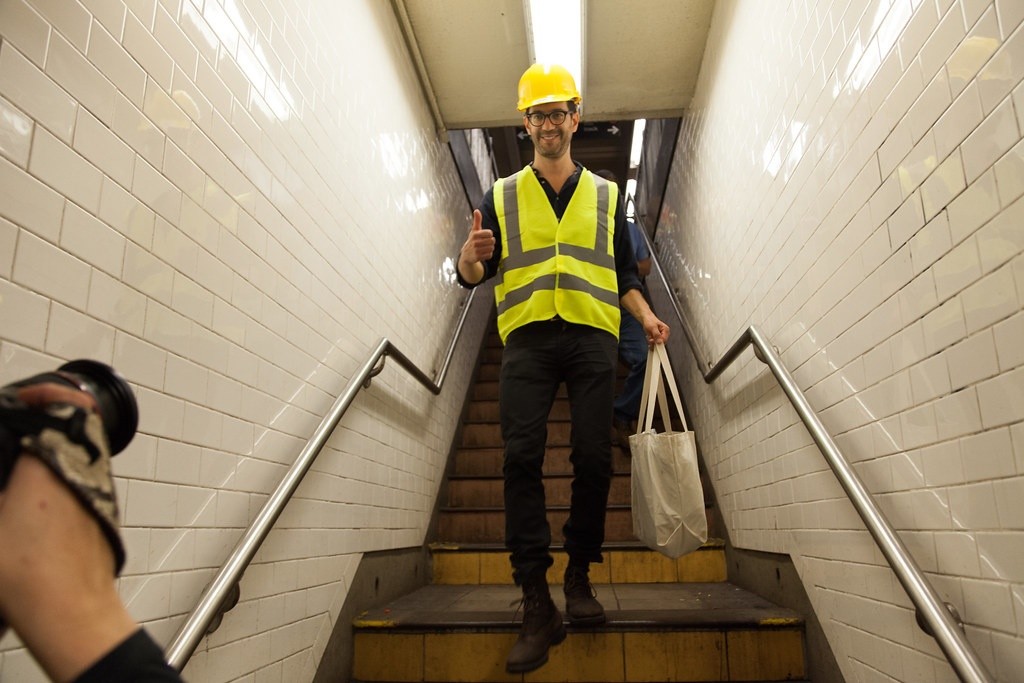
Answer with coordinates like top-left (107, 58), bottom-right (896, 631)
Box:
top-left (518, 62), bottom-right (583, 110)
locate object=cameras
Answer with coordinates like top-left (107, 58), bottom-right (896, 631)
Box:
top-left (0, 358), bottom-right (140, 488)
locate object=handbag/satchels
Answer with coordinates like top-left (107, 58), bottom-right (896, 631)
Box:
top-left (628, 338), bottom-right (708, 558)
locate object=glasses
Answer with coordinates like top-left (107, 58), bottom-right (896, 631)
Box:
top-left (524, 111), bottom-right (575, 127)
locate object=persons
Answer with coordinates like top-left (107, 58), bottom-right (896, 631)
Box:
top-left (596, 170), bottom-right (652, 453)
top-left (0, 384), bottom-right (187, 683)
top-left (455, 63), bottom-right (671, 675)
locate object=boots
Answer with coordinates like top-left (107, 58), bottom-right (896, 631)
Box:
top-left (564, 565), bottom-right (606, 626)
top-left (504, 579), bottom-right (568, 672)
